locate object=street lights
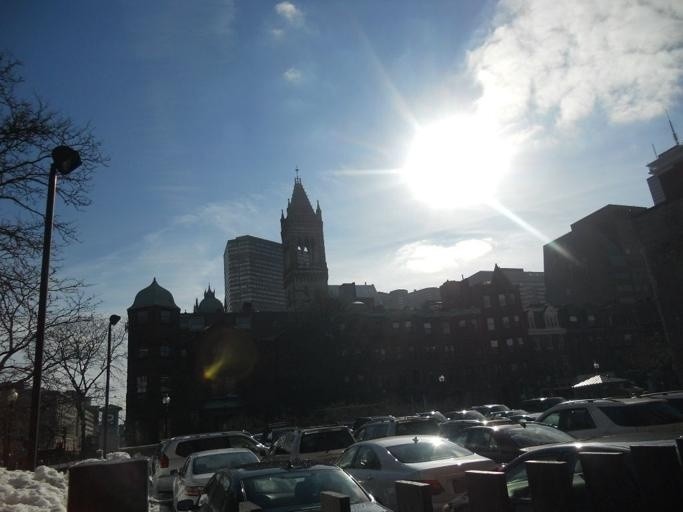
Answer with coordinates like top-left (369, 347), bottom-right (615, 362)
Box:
top-left (3, 388), bottom-right (20, 473)
top-left (99, 312), bottom-right (121, 461)
top-left (437, 374), bottom-right (446, 416)
top-left (17, 146), bottom-right (84, 475)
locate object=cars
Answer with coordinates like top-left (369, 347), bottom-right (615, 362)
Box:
top-left (169, 446), bottom-right (262, 512)
top-left (332, 434), bottom-right (502, 512)
top-left (533, 395), bottom-right (683, 441)
top-left (439, 433), bottom-right (683, 512)
top-left (191, 459), bottom-right (394, 512)
top-left (251, 395), bottom-right (570, 448)
top-left (437, 419), bottom-right (581, 468)
top-left (145, 429), bottom-right (270, 505)
